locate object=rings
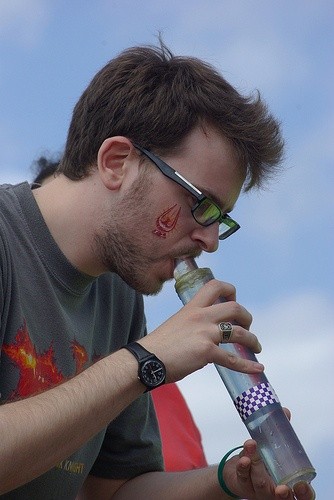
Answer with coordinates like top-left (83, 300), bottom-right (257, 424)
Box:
top-left (218, 319), bottom-right (232, 345)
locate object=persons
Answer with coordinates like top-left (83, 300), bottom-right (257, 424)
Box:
top-left (1, 31), bottom-right (317, 500)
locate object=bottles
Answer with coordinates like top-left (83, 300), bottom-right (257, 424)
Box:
top-left (174, 257), bottom-right (317, 491)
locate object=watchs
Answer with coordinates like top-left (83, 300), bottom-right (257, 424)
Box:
top-left (121, 341), bottom-right (168, 394)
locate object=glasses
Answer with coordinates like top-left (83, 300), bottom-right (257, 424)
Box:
top-left (132, 142), bottom-right (241, 240)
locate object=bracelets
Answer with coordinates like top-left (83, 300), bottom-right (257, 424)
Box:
top-left (218, 441), bottom-right (246, 495)
